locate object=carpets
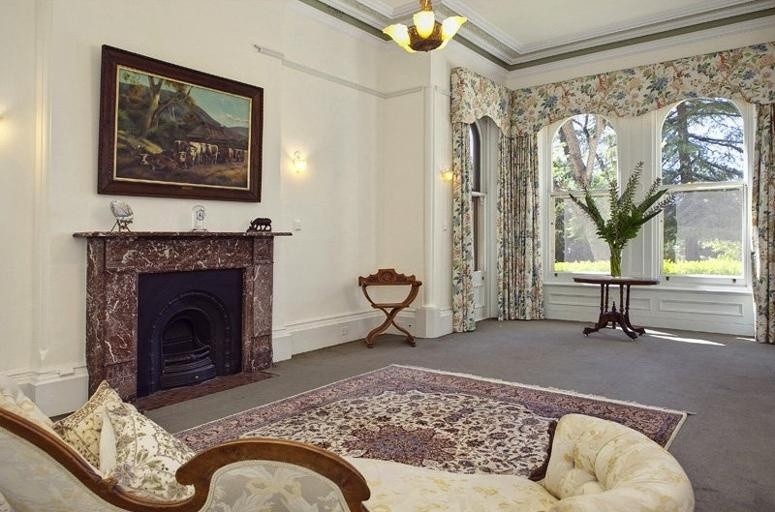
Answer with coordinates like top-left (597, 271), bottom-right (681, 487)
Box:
top-left (170, 361), bottom-right (699, 494)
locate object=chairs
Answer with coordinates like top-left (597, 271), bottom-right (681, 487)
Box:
top-left (355, 266), bottom-right (419, 347)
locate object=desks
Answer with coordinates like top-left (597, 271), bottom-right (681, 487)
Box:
top-left (572, 278), bottom-right (659, 341)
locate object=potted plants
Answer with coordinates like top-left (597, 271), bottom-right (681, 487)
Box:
top-left (566, 160), bottom-right (680, 277)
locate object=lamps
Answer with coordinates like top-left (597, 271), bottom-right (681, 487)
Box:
top-left (292, 151), bottom-right (306, 173)
top-left (380, 1), bottom-right (467, 55)
top-left (441, 167), bottom-right (454, 181)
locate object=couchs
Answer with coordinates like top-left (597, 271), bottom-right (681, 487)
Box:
top-left (0, 377), bottom-right (695, 511)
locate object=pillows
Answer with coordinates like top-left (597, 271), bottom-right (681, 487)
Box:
top-left (53, 379), bottom-right (195, 504)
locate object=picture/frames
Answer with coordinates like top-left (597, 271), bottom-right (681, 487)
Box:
top-left (94, 44), bottom-right (265, 205)
